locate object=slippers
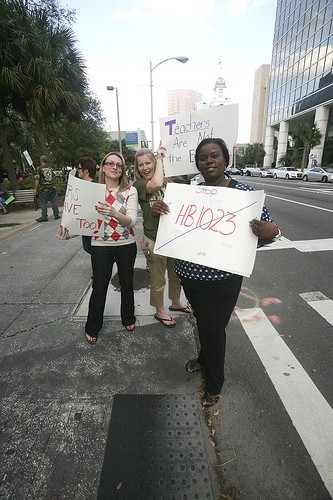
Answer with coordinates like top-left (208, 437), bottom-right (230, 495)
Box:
top-left (154, 313), bottom-right (177, 328)
top-left (169, 305), bottom-right (193, 313)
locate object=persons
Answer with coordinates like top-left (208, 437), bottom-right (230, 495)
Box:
top-left (150, 137), bottom-right (282, 407)
top-left (56, 151), bottom-right (139, 345)
top-left (133, 140), bottom-right (192, 328)
top-left (0, 186), bottom-right (10, 214)
top-left (77, 157), bottom-right (103, 255)
top-left (33, 155), bottom-right (62, 222)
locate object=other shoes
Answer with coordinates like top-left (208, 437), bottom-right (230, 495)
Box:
top-left (36, 217), bottom-right (48, 222)
top-left (54, 214), bottom-right (60, 219)
top-left (2, 208), bottom-right (9, 215)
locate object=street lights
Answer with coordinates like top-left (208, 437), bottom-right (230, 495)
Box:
top-left (106, 85), bottom-right (122, 156)
top-left (149, 55), bottom-right (189, 155)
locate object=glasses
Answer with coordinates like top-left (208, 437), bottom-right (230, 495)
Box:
top-left (77, 167), bottom-right (82, 172)
top-left (104, 160), bottom-right (123, 169)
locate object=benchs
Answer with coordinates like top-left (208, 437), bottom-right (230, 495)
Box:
top-left (7, 189), bottom-right (39, 211)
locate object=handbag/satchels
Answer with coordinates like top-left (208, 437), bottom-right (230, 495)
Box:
top-left (81, 235), bottom-right (96, 255)
top-left (56, 225), bottom-right (71, 240)
top-left (4, 194), bottom-right (16, 205)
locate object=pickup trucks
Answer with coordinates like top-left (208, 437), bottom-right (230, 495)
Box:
top-left (239, 164), bottom-right (260, 176)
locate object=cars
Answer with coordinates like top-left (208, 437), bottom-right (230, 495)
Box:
top-left (226, 166), bottom-right (241, 175)
top-left (250, 167), bottom-right (274, 178)
top-left (301, 166), bottom-right (333, 183)
top-left (273, 166), bottom-right (305, 180)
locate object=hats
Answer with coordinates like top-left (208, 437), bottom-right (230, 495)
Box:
top-left (39, 155), bottom-right (48, 160)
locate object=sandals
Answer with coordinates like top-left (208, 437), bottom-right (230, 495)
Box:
top-left (185, 358), bottom-right (202, 375)
top-left (201, 393), bottom-right (220, 407)
top-left (126, 323), bottom-right (135, 331)
top-left (85, 332), bottom-right (97, 344)
